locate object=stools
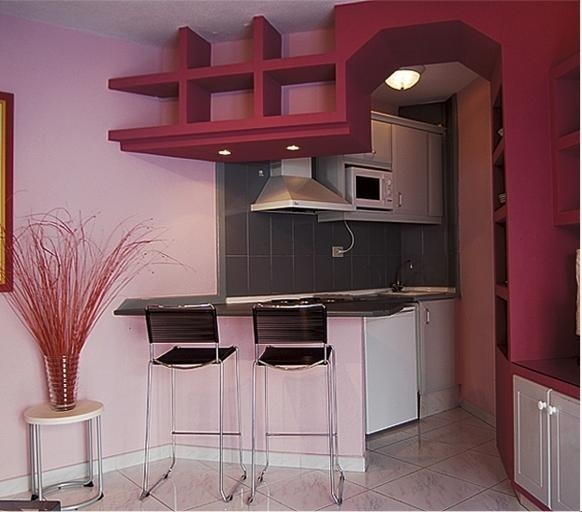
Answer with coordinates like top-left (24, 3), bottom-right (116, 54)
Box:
top-left (21, 399), bottom-right (105, 511)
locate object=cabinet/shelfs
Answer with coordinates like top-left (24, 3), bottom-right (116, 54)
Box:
top-left (417, 299), bottom-right (458, 396)
top-left (486, 64), bottom-right (580, 364)
top-left (512, 373), bottom-right (580, 512)
top-left (548, 49), bottom-right (580, 228)
top-left (315, 118), bottom-right (392, 213)
top-left (392, 122), bottom-right (444, 218)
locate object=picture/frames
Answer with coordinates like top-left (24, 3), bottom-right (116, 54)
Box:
top-left (0, 91), bottom-right (15, 294)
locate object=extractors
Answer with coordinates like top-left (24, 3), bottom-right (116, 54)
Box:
top-left (251, 159), bottom-right (354, 215)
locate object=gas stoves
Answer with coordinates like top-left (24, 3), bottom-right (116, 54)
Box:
top-left (254, 296), bottom-right (395, 310)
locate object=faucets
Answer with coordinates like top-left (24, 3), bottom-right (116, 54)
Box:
top-left (392, 259), bottom-right (413, 293)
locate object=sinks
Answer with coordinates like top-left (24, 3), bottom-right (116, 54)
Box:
top-left (381, 290), bottom-right (447, 295)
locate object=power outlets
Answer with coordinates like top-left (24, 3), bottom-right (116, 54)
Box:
top-left (332, 246), bottom-right (344, 257)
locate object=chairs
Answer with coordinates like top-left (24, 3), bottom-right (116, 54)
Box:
top-left (246, 302), bottom-right (345, 508)
top-left (138, 302), bottom-right (248, 506)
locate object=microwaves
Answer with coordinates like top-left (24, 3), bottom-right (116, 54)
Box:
top-left (344, 165), bottom-right (395, 211)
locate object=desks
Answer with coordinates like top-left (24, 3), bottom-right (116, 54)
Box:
top-left (113, 297), bottom-right (406, 476)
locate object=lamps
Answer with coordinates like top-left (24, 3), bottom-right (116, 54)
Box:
top-left (286, 145), bottom-right (300, 150)
top-left (218, 149), bottom-right (231, 156)
top-left (384, 65), bottom-right (426, 92)
